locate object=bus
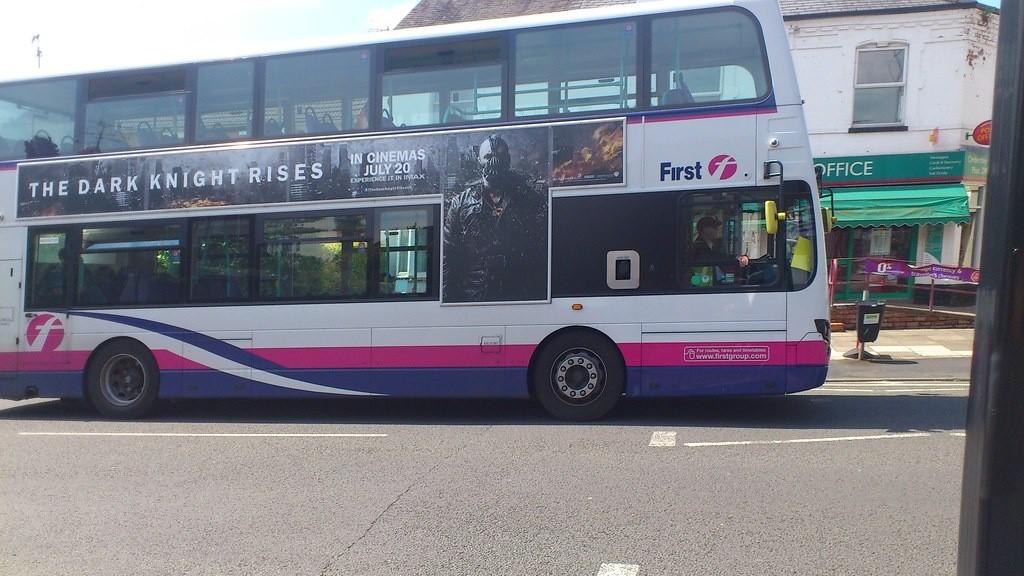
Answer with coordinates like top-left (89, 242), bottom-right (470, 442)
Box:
top-left (1, 0), bottom-right (834, 427)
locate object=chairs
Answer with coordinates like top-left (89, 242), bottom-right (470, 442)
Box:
top-left (32, 106), bottom-right (408, 158)
top-left (83, 265), bottom-right (184, 304)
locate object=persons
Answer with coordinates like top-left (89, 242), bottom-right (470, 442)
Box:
top-left (689, 217), bottom-right (750, 286)
top-left (42, 248), bottom-right (181, 306)
top-left (443, 133), bottom-right (547, 302)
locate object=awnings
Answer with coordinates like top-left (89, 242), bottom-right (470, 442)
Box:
top-left (820, 184), bottom-right (971, 229)
